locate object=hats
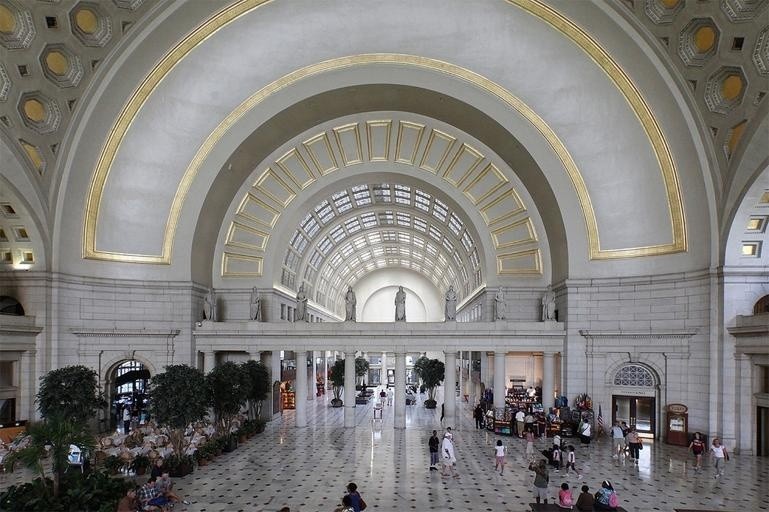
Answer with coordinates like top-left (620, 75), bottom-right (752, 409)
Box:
top-left (445, 432), bottom-right (452, 436)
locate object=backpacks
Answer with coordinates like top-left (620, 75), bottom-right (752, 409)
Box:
top-left (560, 490), bottom-right (572, 506)
top-left (579, 493), bottom-right (593, 510)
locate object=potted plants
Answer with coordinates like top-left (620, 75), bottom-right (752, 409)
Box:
top-left (355, 355), bottom-right (370, 392)
top-left (328, 357), bottom-right (345, 408)
top-left (422, 358), bottom-right (446, 409)
top-left (413, 354), bottom-right (431, 393)
top-left (102, 359), bottom-right (273, 478)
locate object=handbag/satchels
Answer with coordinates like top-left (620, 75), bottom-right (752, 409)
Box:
top-left (638, 440), bottom-right (643, 450)
top-left (724, 451), bottom-right (729, 461)
top-left (360, 499), bottom-right (366, 511)
top-left (609, 493), bottom-right (618, 508)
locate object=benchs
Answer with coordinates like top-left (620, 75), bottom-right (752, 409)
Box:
top-left (529, 502), bottom-right (628, 512)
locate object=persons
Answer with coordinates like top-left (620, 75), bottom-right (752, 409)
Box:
top-left (250, 287), bottom-right (262, 322)
top-left (116, 462), bottom-right (189, 512)
top-left (708, 439), bottom-right (728, 478)
top-left (473, 384), bottom-right (642, 511)
top-left (494, 285), bottom-right (505, 320)
top-left (345, 286), bottom-right (356, 321)
top-left (380, 389), bottom-right (386, 407)
top-left (337, 494), bottom-right (354, 512)
top-left (295, 287), bottom-right (309, 322)
top-left (386, 388), bottom-right (393, 406)
top-left (394, 286), bottom-right (406, 322)
top-left (348, 482), bottom-right (361, 512)
top-left (429, 426), bottom-right (460, 478)
top-left (112, 389), bottom-right (153, 435)
top-left (541, 284), bottom-right (556, 321)
top-left (445, 285), bottom-right (456, 320)
top-left (205, 287), bottom-right (218, 321)
top-left (406, 383), bottom-right (418, 404)
top-left (688, 432), bottom-right (706, 471)
